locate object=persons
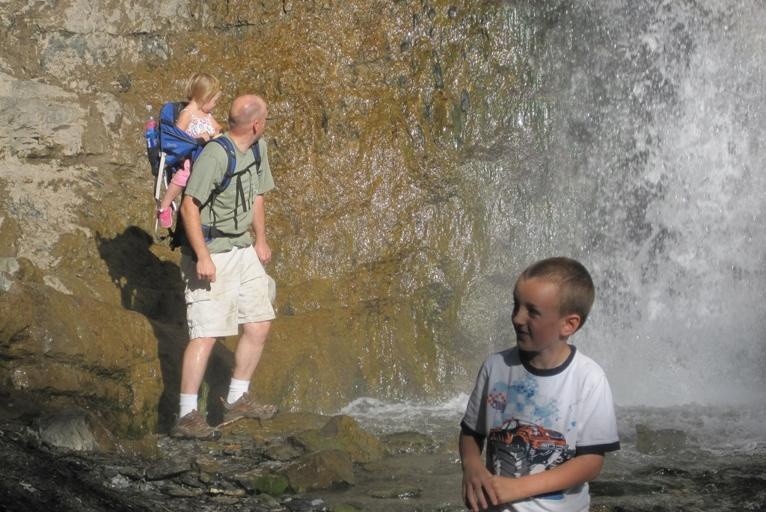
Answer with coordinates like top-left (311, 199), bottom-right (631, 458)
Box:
top-left (158, 71), bottom-right (223, 231)
top-left (458, 256), bottom-right (621, 512)
top-left (167, 95), bottom-right (279, 441)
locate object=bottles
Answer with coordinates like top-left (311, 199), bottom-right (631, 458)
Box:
top-left (144, 118), bottom-right (158, 149)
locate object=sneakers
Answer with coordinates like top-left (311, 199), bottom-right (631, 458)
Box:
top-left (169, 411), bottom-right (221, 442)
top-left (159, 207), bottom-right (174, 229)
top-left (219, 393), bottom-right (279, 421)
